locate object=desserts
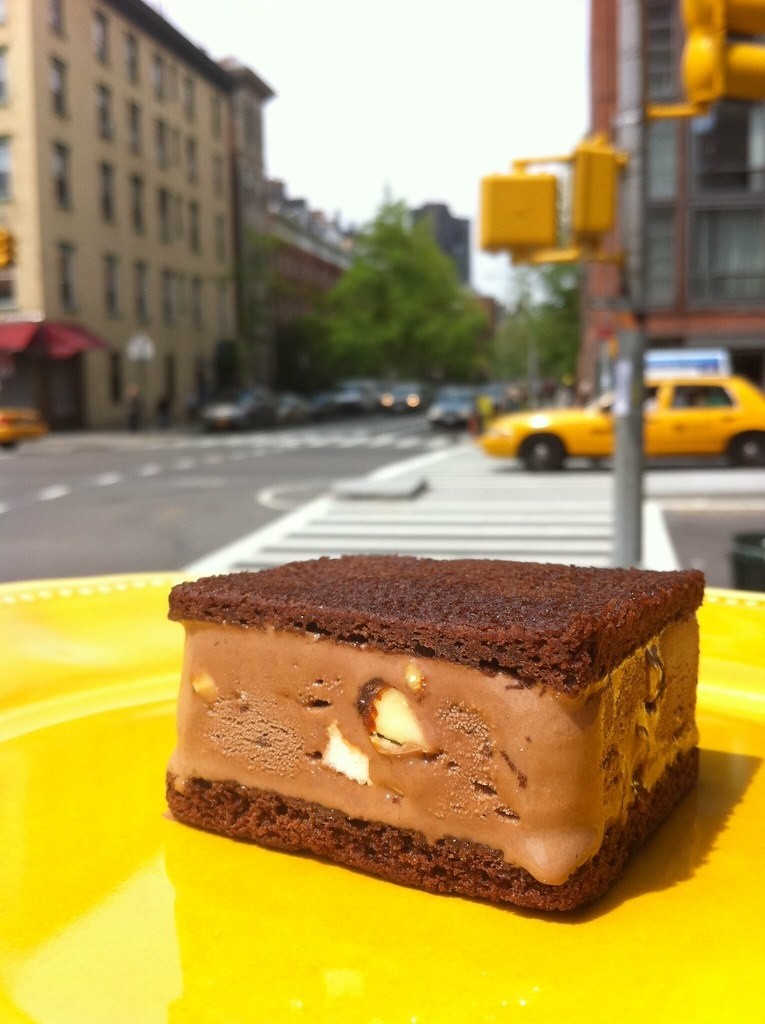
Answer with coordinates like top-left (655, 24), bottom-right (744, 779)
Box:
top-left (166, 553), bottom-right (706, 910)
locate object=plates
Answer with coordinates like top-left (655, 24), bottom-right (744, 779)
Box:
top-left (0, 570), bottom-right (765, 1024)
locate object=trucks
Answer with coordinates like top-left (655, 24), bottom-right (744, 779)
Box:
top-left (616, 348), bottom-right (733, 379)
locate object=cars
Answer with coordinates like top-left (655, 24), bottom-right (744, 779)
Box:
top-left (196, 377), bottom-right (549, 434)
top-left (477, 369), bottom-right (765, 470)
top-left (0, 407), bottom-right (47, 448)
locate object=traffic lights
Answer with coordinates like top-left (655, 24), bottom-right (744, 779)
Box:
top-left (675, 0), bottom-right (765, 111)
top-left (1, 229), bottom-right (13, 269)
